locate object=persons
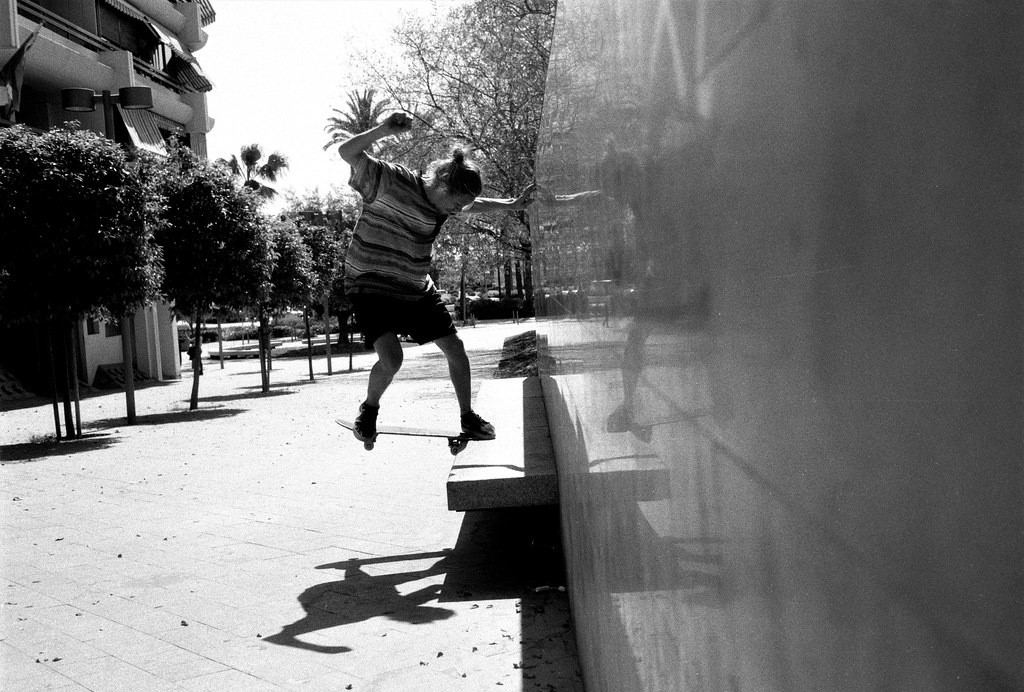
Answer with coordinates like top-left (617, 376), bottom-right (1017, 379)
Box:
top-left (537, 134), bottom-right (708, 435)
top-left (185, 330), bottom-right (204, 375)
top-left (338, 110), bottom-right (535, 441)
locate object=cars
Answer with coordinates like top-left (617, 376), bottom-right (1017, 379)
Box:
top-left (437, 289), bottom-right (501, 304)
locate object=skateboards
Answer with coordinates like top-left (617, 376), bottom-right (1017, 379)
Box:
top-left (606, 405), bottom-right (717, 443)
top-left (335, 417), bottom-right (497, 455)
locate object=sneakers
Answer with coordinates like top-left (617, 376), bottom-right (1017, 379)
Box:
top-left (354, 401), bottom-right (379, 442)
top-left (461, 415), bottom-right (494, 435)
